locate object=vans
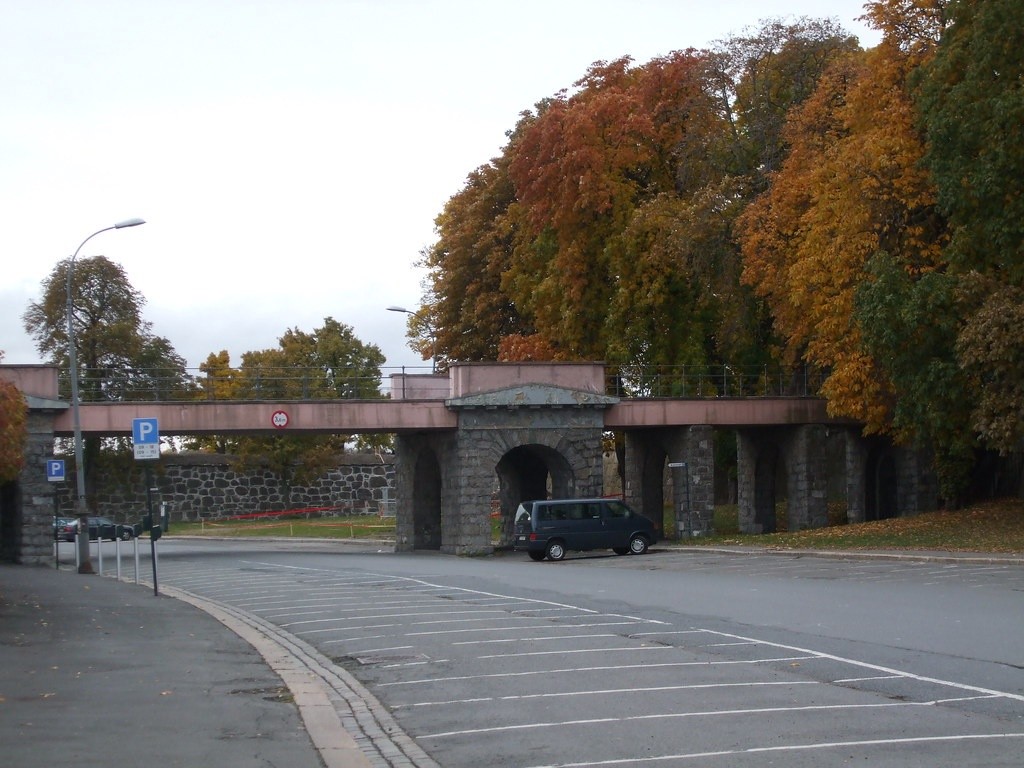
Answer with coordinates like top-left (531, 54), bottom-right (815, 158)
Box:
top-left (512, 499), bottom-right (657, 563)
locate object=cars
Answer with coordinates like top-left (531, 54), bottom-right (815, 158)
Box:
top-left (64, 517), bottom-right (135, 541)
top-left (53, 516), bottom-right (74, 540)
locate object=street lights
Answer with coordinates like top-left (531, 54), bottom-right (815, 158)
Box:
top-left (386, 306), bottom-right (436, 375)
top-left (66, 219), bottom-right (147, 577)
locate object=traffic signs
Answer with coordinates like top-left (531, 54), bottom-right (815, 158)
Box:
top-left (133, 417), bottom-right (161, 460)
